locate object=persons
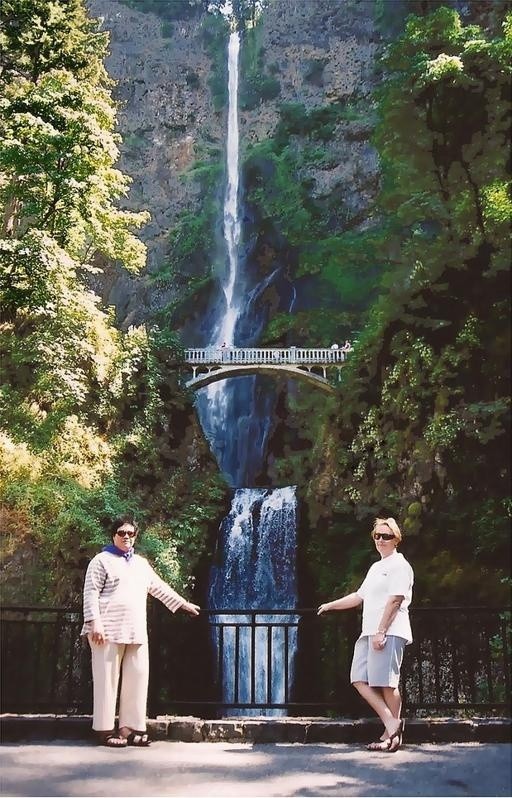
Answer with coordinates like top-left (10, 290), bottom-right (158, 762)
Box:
top-left (222, 342), bottom-right (227, 348)
top-left (331, 339), bottom-right (351, 351)
top-left (78, 516), bottom-right (201, 747)
top-left (317, 518), bottom-right (413, 752)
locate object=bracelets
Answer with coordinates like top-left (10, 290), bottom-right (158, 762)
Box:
top-left (377, 631), bottom-right (384, 634)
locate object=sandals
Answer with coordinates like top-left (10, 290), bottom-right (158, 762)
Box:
top-left (364, 736), bottom-right (386, 751)
top-left (386, 717), bottom-right (405, 753)
top-left (119, 730), bottom-right (152, 746)
top-left (97, 733), bottom-right (129, 747)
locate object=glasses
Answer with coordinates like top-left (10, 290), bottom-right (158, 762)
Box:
top-left (374, 533), bottom-right (395, 540)
top-left (116, 531), bottom-right (135, 537)
